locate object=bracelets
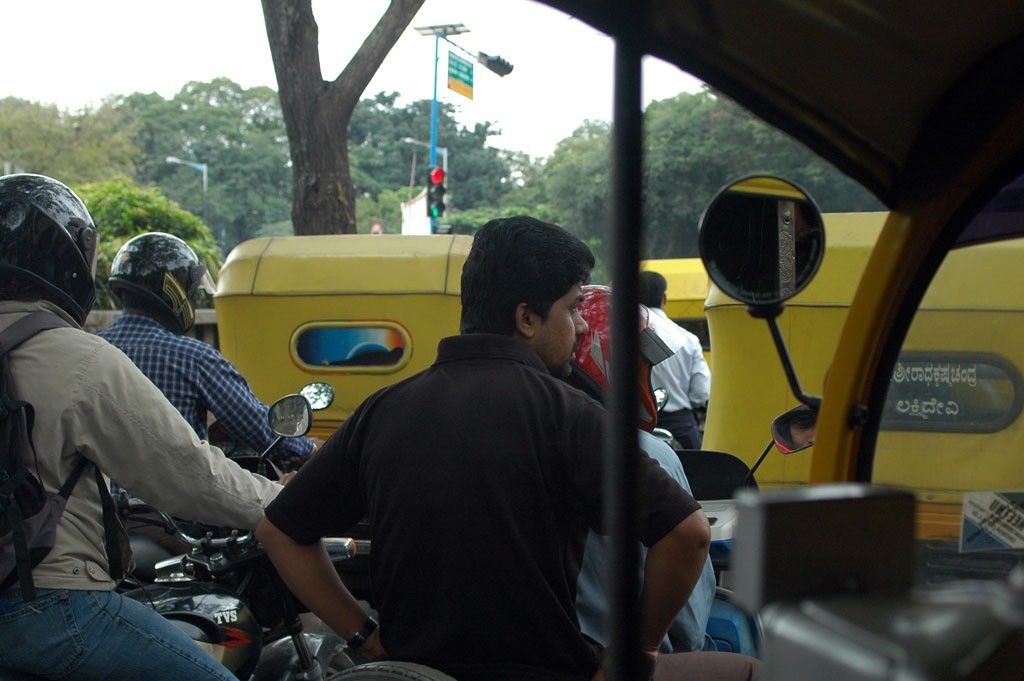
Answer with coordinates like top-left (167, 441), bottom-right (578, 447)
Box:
top-left (347, 616), bottom-right (377, 650)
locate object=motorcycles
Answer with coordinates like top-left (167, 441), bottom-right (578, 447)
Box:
top-left (631, 210), bottom-right (1022, 657)
top-left (112, 381), bottom-right (462, 681)
top-left (213, 230), bottom-right (473, 453)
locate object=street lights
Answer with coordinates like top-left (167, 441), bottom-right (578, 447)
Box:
top-left (165, 156), bottom-right (208, 191)
top-left (404, 136), bottom-right (449, 188)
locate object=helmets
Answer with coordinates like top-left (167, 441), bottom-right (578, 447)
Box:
top-left (110, 231), bottom-right (206, 332)
top-left (0, 173), bottom-right (100, 328)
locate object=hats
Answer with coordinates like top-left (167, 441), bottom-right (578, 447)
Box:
top-left (636, 271), bottom-right (668, 294)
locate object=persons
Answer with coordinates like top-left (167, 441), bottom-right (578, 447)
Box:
top-left (255, 215), bottom-right (762, 681)
top-left (788, 411), bottom-right (816, 448)
top-left (0, 173), bottom-right (298, 680)
top-left (637, 271), bottom-right (711, 449)
top-left (100, 232), bottom-right (317, 610)
top-left (561, 285), bottom-right (717, 655)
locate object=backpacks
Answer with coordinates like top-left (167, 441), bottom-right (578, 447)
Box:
top-left (0, 312), bottom-right (86, 594)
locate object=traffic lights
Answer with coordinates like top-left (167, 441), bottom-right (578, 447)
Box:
top-left (426, 165), bottom-right (445, 218)
top-left (486, 54), bottom-right (514, 77)
